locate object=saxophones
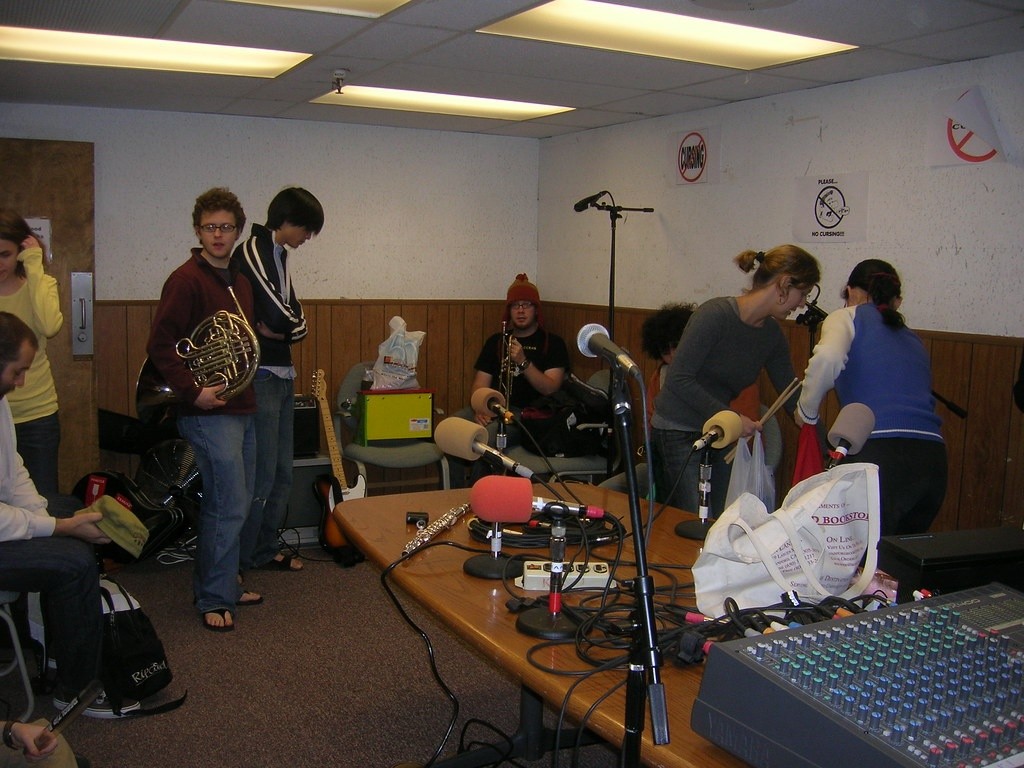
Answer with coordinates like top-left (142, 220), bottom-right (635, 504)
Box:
top-left (496, 320), bottom-right (517, 423)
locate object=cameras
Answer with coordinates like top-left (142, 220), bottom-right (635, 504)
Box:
top-left (406, 511), bottom-right (429, 528)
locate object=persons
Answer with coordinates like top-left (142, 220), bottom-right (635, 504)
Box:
top-left (596, 307), bottom-right (764, 521)
top-left (795, 260), bottom-right (949, 570)
top-left (227, 187), bottom-right (324, 588)
top-left (648, 246), bottom-right (821, 523)
top-left (146, 187), bottom-right (263, 633)
top-left (440, 273), bottom-right (569, 489)
top-left (0, 720), bottom-right (78, 768)
top-left (0, 311), bottom-right (142, 719)
top-left (0, 204), bottom-right (64, 505)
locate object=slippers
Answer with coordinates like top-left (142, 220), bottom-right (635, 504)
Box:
top-left (235, 591), bottom-right (264, 605)
top-left (259, 555), bottom-right (304, 571)
top-left (202, 609), bottom-right (234, 632)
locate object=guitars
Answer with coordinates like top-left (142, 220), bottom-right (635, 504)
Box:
top-left (308, 367), bottom-right (369, 557)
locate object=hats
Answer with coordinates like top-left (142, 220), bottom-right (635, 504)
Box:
top-left (73, 495), bottom-right (149, 559)
top-left (498, 273), bottom-right (548, 368)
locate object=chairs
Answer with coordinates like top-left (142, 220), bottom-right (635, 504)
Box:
top-left (334, 359), bottom-right (450, 498)
top-left (501, 360), bottom-right (634, 485)
top-left (760, 404), bottom-right (782, 475)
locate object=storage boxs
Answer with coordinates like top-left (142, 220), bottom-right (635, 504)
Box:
top-left (353, 389), bottom-right (434, 447)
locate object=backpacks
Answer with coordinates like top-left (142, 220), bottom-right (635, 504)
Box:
top-left (99, 576), bottom-right (187, 716)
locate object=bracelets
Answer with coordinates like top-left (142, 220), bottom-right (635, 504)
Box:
top-left (3, 719), bottom-right (22, 752)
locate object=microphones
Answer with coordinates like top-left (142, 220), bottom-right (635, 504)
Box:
top-left (470, 388), bottom-right (520, 420)
top-left (578, 324), bottom-right (641, 377)
top-left (573, 191), bottom-right (609, 213)
top-left (692, 411), bottom-right (743, 451)
top-left (471, 474), bottom-right (605, 523)
top-left (825, 402), bottom-right (875, 473)
top-left (433, 417), bottom-right (534, 478)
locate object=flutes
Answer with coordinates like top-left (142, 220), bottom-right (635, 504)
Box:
top-left (402, 503), bottom-right (472, 561)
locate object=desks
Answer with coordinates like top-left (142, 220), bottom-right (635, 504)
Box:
top-left (332, 481), bottom-right (854, 768)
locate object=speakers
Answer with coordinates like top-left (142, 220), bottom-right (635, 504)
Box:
top-left (293, 393), bottom-right (320, 459)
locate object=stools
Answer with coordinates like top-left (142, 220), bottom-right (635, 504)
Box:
top-left (0, 590), bottom-right (35, 726)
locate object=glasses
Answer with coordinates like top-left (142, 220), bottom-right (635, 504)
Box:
top-left (509, 302), bottom-right (536, 310)
top-left (198, 223), bottom-right (238, 233)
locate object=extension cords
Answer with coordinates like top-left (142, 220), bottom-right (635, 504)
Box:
top-left (514, 561), bottom-right (617, 592)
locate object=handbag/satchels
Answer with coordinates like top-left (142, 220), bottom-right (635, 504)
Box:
top-left (70, 469), bottom-right (189, 566)
top-left (724, 430), bottom-right (775, 514)
top-left (691, 462), bottom-right (880, 623)
top-left (370, 316), bottom-right (426, 390)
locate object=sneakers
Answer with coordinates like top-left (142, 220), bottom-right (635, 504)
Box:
top-left (52, 682), bottom-right (141, 719)
top-left (48, 649), bottom-right (57, 669)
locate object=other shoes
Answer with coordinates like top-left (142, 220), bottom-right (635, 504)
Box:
top-left (74, 754), bottom-right (89, 768)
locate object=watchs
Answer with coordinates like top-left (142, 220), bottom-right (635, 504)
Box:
top-left (518, 358), bottom-right (531, 373)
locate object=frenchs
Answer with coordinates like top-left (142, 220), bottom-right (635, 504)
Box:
top-left (134, 286), bottom-right (261, 433)
